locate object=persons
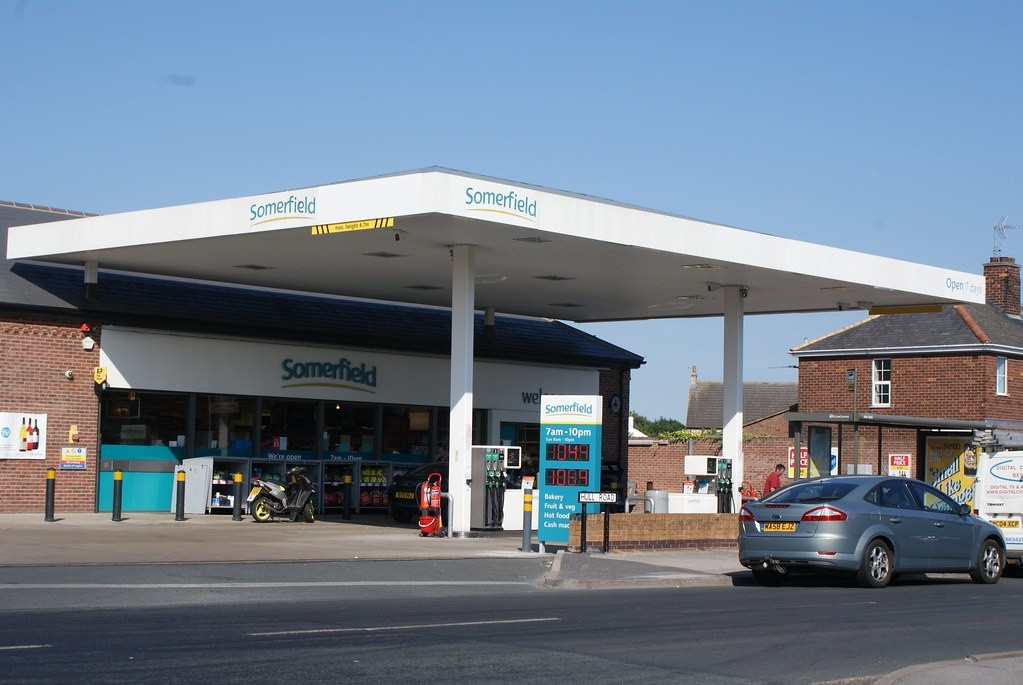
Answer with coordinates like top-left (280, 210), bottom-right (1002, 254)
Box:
top-left (763, 463), bottom-right (786, 497)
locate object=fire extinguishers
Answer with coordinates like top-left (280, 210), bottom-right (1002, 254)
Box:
top-left (415, 480), bottom-right (431, 510)
top-left (429, 481), bottom-right (441, 509)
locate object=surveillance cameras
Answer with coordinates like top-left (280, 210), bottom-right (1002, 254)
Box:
top-left (65, 370), bottom-right (73, 379)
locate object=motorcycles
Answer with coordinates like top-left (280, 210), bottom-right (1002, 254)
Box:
top-left (246, 464), bottom-right (317, 523)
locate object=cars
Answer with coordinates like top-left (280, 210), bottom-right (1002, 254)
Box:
top-left (738, 474), bottom-right (1007, 589)
top-left (388, 460), bottom-right (449, 528)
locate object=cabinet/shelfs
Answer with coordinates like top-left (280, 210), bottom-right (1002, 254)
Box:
top-left (182, 455), bottom-right (430, 515)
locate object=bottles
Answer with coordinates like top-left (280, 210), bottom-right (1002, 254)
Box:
top-left (26, 418), bottom-right (33, 450)
top-left (18, 417), bottom-right (27, 452)
top-left (33, 419), bottom-right (39, 449)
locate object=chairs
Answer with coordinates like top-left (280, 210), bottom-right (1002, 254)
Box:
top-left (885, 490), bottom-right (907, 508)
